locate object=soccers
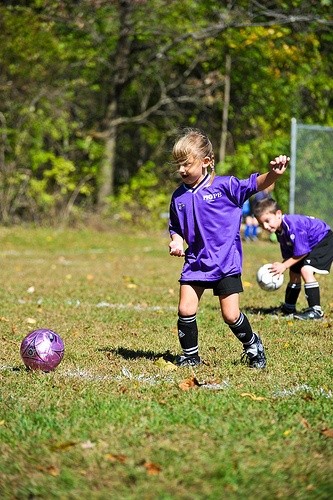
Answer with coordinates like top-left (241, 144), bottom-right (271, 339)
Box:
top-left (257, 264), bottom-right (284, 291)
top-left (20, 329), bottom-right (64, 372)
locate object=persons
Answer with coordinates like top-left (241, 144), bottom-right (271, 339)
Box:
top-left (168, 128), bottom-right (290, 371)
top-left (253, 197), bottom-right (333, 321)
top-left (241, 177), bottom-right (276, 243)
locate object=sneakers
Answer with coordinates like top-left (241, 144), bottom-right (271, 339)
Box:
top-left (176, 356), bottom-right (200, 366)
top-left (241, 333), bottom-right (266, 368)
top-left (294, 307), bottom-right (324, 320)
top-left (272, 304), bottom-right (296, 316)
